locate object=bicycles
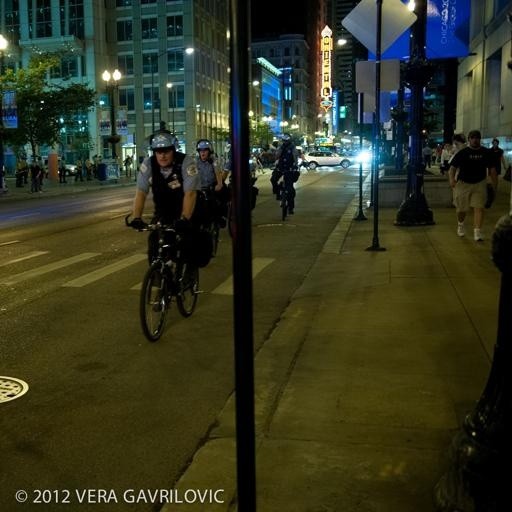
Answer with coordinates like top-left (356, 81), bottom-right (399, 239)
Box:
top-left (126, 174), bottom-right (291, 344)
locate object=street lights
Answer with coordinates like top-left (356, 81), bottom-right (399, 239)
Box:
top-left (165, 81), bottom-right (176, 138)
top-left (102, 68), bottom-right (123, 158)
top-left (150, 46), bottom-right (196, 135)
top-left (261, 114), bottom-right (274, 146)
top-left (279, 121), bottom-right (289, 134)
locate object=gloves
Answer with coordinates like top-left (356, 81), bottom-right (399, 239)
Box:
top-left (131, 217), bottom-right (145, 228)
top-left (172, 217), bottom-right (189, 232)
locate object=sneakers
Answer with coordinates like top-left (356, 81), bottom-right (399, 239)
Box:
top-left (276, 193), bottom-right (282, 201)
top-left (456, 221), bottom-right (465, 237)
top-left (288, 207), bottom-right (294, 215)
top-left (473, 228), bottom-right (484, 242)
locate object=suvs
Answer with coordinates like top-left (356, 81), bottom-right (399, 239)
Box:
top-left (26, 155), bottom-right (77, 177)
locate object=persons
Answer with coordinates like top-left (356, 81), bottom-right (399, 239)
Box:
top-left (422, 128), bottom-right (506, 190)
top-left (446, 131), bottom-right (498, 241)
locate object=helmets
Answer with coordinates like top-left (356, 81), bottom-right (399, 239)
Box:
top-left (197, 140), bottom-right (213, 152)
top-left (150, 132), bottom-right (178, 149)
top-left (282, 134), bottom-right (291, 140)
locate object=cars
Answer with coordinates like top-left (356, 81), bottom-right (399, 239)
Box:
top-left (249, 142), bottom-right (371, 169)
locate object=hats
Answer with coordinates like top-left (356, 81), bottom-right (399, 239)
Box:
top-left (468, 130), bottom-right (481, 139)
